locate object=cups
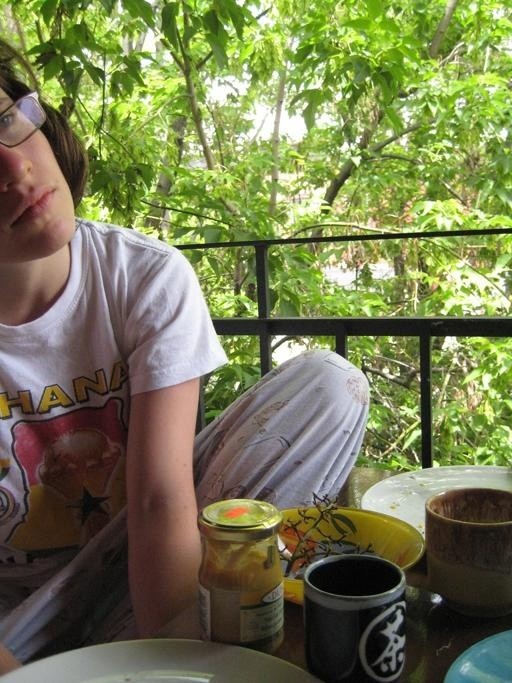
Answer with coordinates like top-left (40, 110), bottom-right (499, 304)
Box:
top-left (417, 476), bottom-right (512, 612)
top-left (307, 553), bottom-right (408, 681)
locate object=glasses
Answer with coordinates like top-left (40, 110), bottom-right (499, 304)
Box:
top-left (0, 91), bottom-right (49, 149)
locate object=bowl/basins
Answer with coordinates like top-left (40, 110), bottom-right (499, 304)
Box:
top-left (270, 507), bottom-right (421, 611)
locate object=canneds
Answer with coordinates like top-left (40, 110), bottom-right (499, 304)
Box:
top-left (196, 498), bottom-right (286, 655)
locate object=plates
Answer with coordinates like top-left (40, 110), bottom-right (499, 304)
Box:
top-left (360, 465), bottom-right (512, 531)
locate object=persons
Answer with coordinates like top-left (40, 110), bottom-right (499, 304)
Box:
top-left (0, 33), bottom-right (370, 679)
